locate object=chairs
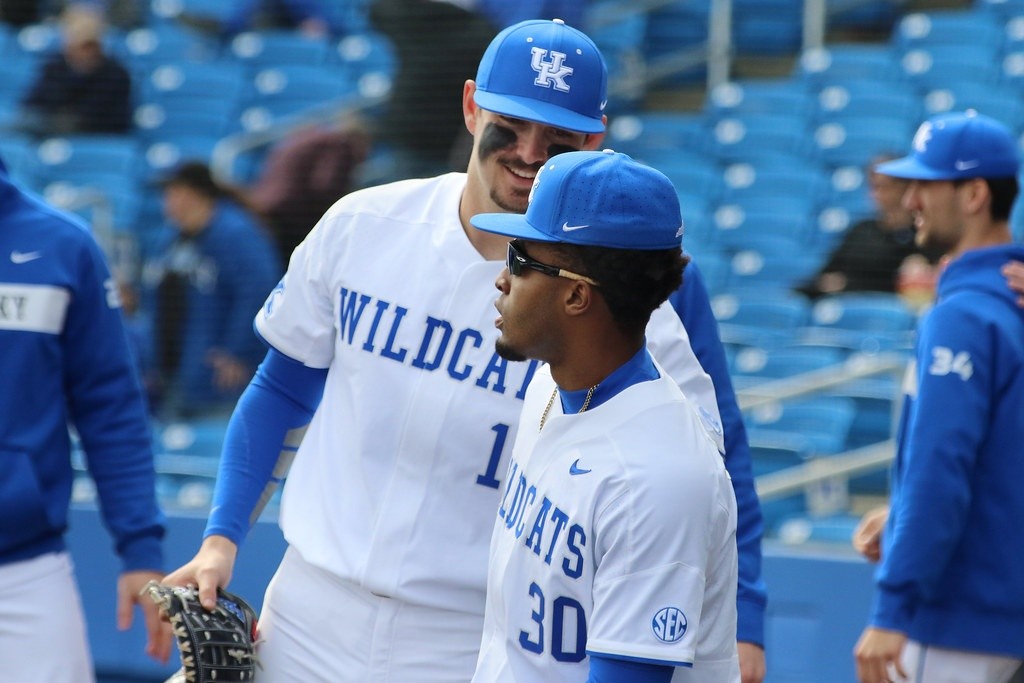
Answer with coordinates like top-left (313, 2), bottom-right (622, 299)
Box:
top-left (0, 0), bottom-right (1024, 549)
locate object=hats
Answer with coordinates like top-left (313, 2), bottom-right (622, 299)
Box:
top-left (475, 18), bottom-right (610, 135)
top-left (874, 109), bottom-right (1023, 181)
top-left (470, 149), bottom-right (683, 250)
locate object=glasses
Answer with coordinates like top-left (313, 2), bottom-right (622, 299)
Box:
top-left (505, 238), bottom-right (602, 288)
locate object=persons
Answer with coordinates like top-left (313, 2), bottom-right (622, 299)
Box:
top-left (119, 0), bottom-right (500, 420)
top-left (465, 148), bottom-right (742, 681)
top-left (805, 107), bottom-right (1023, 683)
top-left (25, 6), bottom-right (138, 140)
top-left (0, 151), bottom-right (175, 682)
top-left (161, 19), bottom-right (769, 682)
